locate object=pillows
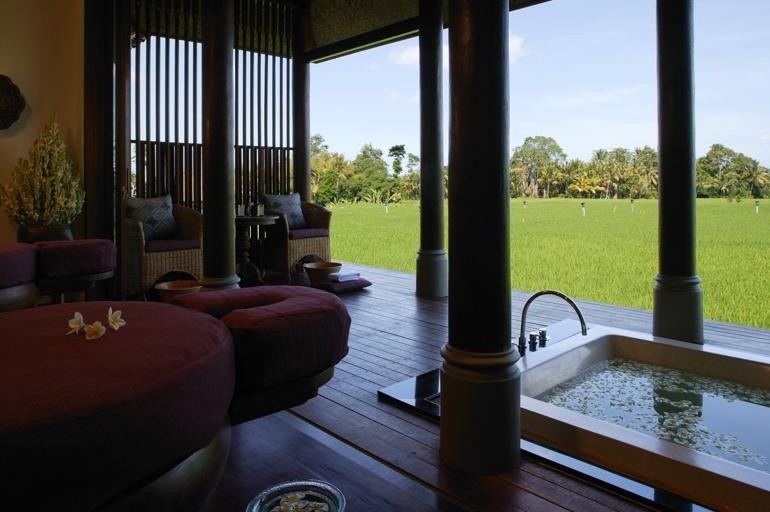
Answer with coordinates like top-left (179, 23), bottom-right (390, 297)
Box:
top-left (126, 194), bottom-right (179, 240)
top-left (261, 192), bottom-right (305, 227)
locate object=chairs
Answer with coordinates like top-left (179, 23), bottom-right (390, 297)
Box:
top-left (265, 201), bottom-right (333, 273)
top-left (172, 285), bottom-right (351, 426)
top-left (123, 205), bottom-right (204, 302)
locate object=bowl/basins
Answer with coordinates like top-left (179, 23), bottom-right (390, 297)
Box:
top-left (303, 261), bottom-right (342, 283)
top-left (246, 478), bottom-right (346, 512)
top-left (153, 278), bottom-right (203, 303)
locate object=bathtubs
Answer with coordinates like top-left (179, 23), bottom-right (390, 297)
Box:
top-left (519, 325), bottom-right (769, 512)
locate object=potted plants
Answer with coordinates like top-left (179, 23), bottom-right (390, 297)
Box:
top-left (1, 111), bottom-right (86, 243)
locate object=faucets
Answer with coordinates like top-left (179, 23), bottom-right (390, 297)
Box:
top-left (521, 290), bottom-right (587, 340)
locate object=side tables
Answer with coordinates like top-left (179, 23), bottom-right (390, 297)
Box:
top-left (236, 215), bottom-right (280, 282)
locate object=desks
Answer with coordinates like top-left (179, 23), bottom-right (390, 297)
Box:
top-left (0, 239), bottom-right (116, 314)
top-left (0, 299), bottom-right (232, 510)
